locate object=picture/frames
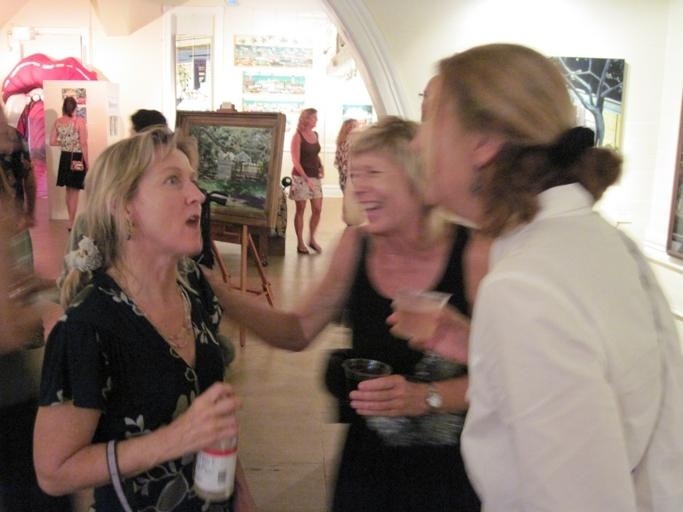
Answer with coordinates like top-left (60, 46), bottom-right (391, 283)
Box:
top-left (175, 110), bottom-right (286, 231)
top-left (665, 90), bottom-right (683, 259)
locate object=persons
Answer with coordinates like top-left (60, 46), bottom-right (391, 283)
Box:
top-left (288, 108), bottom-right (326, 255)
top-left (333, 118), bottom-right (361, 196)
top-left (127, 108), bottom-right (167, 137)
top-left (385, 42), bottom-right (683, 512)
top-left (49, 96), bottom-right (90, 232)
top-left (30, 129), bottom-right (265, 512)
top-left (200, 115), bottom-right (491, 512)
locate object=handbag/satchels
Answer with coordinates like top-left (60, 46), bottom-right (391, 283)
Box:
top-left (70, 160), bottom-right (84, 172)
top-left (343, 176), bottom-right (366, 225)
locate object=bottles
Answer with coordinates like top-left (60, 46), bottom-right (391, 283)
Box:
top-left (192, 433), bottom-right (238, 502)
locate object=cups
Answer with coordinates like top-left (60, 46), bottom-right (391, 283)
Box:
top-left (342, 358), bottom-right (393, 413)
top-left (394, 286), bottom-right (452, 340)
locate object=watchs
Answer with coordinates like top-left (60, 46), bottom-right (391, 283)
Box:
top-left (425, 381), bottom-right (444, 413)
